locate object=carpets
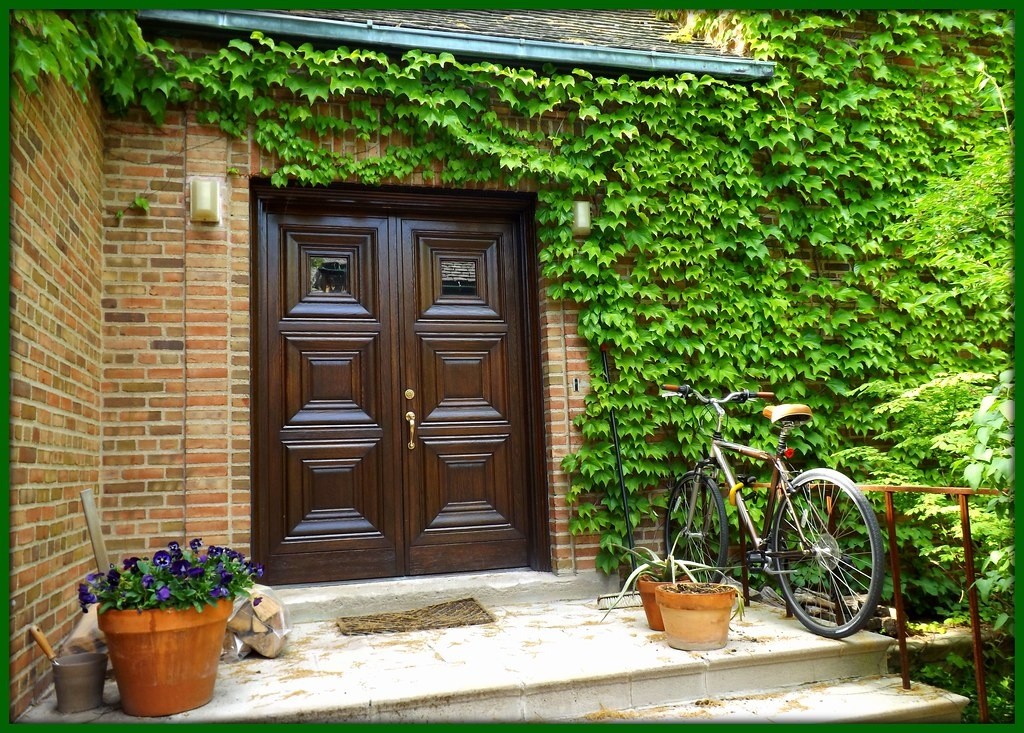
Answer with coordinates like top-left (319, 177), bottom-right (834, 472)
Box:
top-left (335, 597), bottom-right (495, 636)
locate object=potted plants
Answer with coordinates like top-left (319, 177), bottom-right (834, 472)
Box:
top-left (596, 531), bottom-right (745, 631)
top-left (655, 582), bottom-right (736, 651)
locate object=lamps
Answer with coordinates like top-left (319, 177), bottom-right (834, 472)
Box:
top-left (189, 177), bottom-right (220, 222)
top-left (570, 201), bottom-right (590, 236)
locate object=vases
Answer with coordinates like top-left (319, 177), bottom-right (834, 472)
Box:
top-left (97, 596), bottom-right (233, 718)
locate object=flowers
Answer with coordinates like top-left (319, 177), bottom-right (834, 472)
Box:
top-left (78, 537), bottom-right (264, 616)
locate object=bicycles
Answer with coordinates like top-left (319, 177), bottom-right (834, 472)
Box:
top-left (659, 382), bottom-right (885, 639)
